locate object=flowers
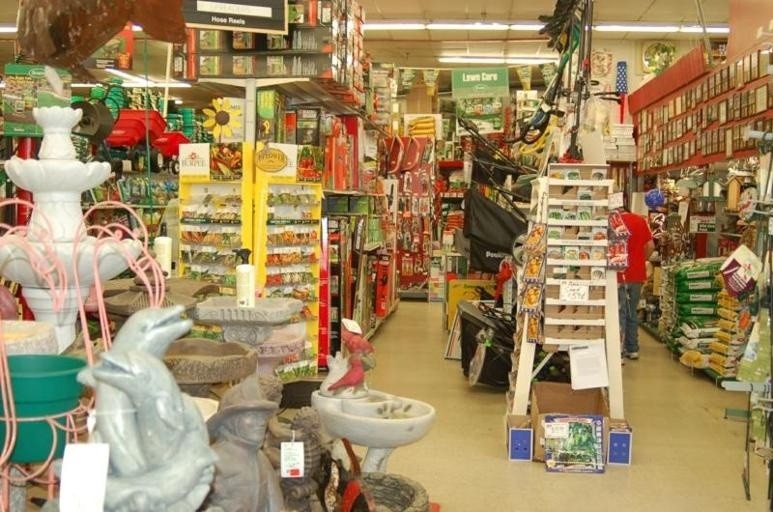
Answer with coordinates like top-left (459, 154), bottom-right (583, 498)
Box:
top-left (202, 98), bottom-right (241, 142)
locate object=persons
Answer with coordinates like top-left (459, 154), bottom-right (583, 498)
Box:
top-left (204, 373), bottom-right (285, 512)
top-left (617, 204), bottom-right (654, 364)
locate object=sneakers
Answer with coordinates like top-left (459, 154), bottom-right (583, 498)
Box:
top-left (621, 353), bottom-right (625, 366)
top-left (622, 349), bottom-right (639, 359)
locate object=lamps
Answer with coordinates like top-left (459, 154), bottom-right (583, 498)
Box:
top-left (595, 26), bottom-right (678, 35)
top-left (426, 24), bottom-right (509, 31)
top-left (512, 25), bottom-right (590, 32)
top-left (364, 23), bottom-right (425, 31)
top-left (153, 83), bottom-right (191, 88)
top-left (0, 27), bottom-right (16, 32)
top-left (122, 83), bottom-right (150, 86)
top-left (506, 59), bottom-right (558, 64)
top-left (436, 59), bottom-right (506, 65)
top-left (105, 67), bottom-right (155, 88)
top-left (682, 24), bottom-right (730, 35)
top-left (71, 83), bottom-right (97, 87)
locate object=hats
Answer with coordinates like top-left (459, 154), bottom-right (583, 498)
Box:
top-left (206, 381), bottom-right (278, 434)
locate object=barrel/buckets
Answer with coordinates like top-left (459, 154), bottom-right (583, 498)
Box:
top-left (0, 355), bottom-right (89, 464)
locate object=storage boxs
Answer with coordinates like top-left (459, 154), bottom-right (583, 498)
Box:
top-left (530, 382), bottom-right (608, 462)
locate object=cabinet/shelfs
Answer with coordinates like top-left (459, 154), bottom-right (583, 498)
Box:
top-left (640, 156), bottom-right (773, 390)
top-left (253, 142), bottom-right (321, 382)
top-left (196, 78), bottom-right (399, 371)
top-left (398, 137), bottom-right (433, 297)
top-left (503, 176), bottom-right (623, 419)
top-left (177, 142), bottom-right (253, 295)
